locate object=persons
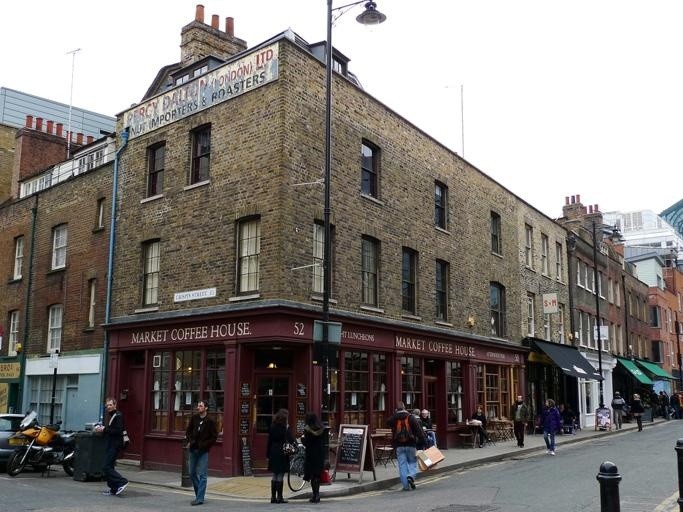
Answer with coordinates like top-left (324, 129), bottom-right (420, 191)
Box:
top-left (539, 398), bottom-right (560, 456)
top-left (300, 411), bottom-right (325, 503)
top-left (386, 401), bottom-right (433, 490)
top-left (470, 405), bottom-right (492, 448)
top-left (265, 408), bottom-right (298, 503)
top-left (185, 400), bottom-right (218, 505)
top-left (95, 394), bottom-right (130, 496)
top-left (509, 395), bottom-right (532, 448)
top-left (612, 389), bottom-right (680, 432)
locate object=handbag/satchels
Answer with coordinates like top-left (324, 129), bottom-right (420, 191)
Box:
top-left (417, 446), bottom-right (445, 471)
top-left (320, 469), bottom-right (329, 482)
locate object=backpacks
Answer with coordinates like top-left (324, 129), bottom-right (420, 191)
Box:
top-left (393, 415), bottom-right (415, 443)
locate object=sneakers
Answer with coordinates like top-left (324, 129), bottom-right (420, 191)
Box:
top-left (115, 482), bottom-right (128, 494)
top-left (191, 501), bottom-right (202, 505)
top-left (546, 449), bottom-right (555, 456)
top-left (103, 491), bottom-right (114, 495)
top-left (407, 477), bottom-right (415, 489)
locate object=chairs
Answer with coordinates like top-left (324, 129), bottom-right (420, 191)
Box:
top-left (365, 425), bottom-right (437, 467)
top-left (460, 415), bottom-right (581, 448)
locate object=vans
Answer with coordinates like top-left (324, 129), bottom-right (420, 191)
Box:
top-left (0, 412), bottom-right (45, 472)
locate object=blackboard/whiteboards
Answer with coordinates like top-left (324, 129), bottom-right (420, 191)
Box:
top-left (295, 383), bottom-right (308, 439)
top-left (241, 438), bottom-right (253, 476)
top-left (334, 424), bottom-right (368, 474)
top-left (239, 380), bottom-right (252, 436)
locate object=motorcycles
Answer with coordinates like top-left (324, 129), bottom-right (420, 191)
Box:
top-left (6, 410), bottom-right (77, 477)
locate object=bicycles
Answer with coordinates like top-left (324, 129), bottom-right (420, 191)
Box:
top-left (285, 428), bottom-right (337, 492)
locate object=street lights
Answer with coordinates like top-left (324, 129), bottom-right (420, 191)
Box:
top-left (590, 219), bottom-right (624, 431)
top-left (319, 0), bottom-right (389, 470)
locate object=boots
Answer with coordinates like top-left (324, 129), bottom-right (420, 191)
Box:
top-left (271, 480), bottom-right (277, 503)
top-left (308, 480), bottom-right (322, 503)
top-left (276, 481), bottom-right (287, 502)
